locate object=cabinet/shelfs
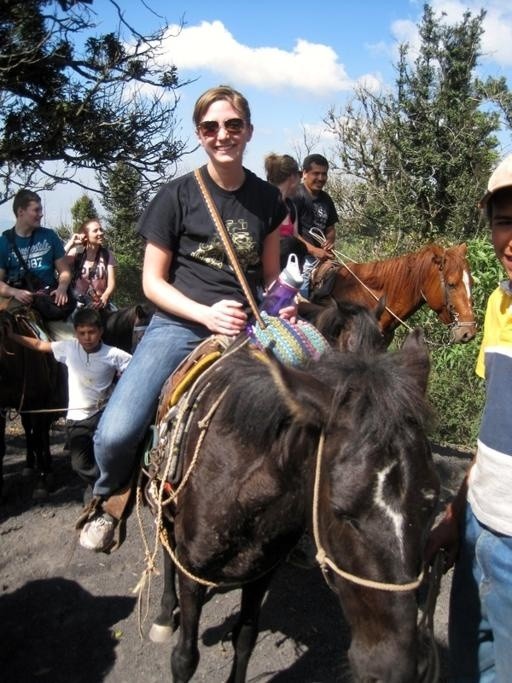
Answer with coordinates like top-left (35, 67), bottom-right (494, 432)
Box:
top-left (244, 252), bottom-right (303, 337)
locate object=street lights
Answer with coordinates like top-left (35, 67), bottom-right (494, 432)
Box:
top-left (1, 226), bottom-right (76, 320)
top-left (191, 165), bottom-right (334, 374)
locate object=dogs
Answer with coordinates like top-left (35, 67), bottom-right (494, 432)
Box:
top-left (296, 238), bottom-right (480, 346)
top-left (147, 332), bottom-right (442, 682)
top-left (98, 301), bottom-right (155, 356)
top-left (0, 306), bottom-right (81, 500)
top-left (296, 292), bottom-right (390, 358)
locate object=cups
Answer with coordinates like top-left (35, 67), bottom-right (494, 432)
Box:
top-left (195, 117), bottom-right (247, 133)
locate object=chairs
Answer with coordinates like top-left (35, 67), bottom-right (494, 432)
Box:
top-left (77, 510), bottom-right (116, 551)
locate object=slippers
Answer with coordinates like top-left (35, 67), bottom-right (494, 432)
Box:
top-left (68, 242), bottom-right (112, 281)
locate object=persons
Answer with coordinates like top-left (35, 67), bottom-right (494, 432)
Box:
top-left (292, 153), bottom-right (339, 299)
top-left (76, 84), bottom-right (301, 552)
top-left (1, 187), bottom-right (80, 342)
top-left (422, 154), bottom-right (512, 659)
top-left (62, 218), bottom-right (118, 316)
top-left (260, 151), bottom-right (305, 293)
top-left (3, 308), bottom-right (133, 521)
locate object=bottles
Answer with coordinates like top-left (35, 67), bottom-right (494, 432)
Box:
top-left (477, 151), bottom-right (512, 208)
